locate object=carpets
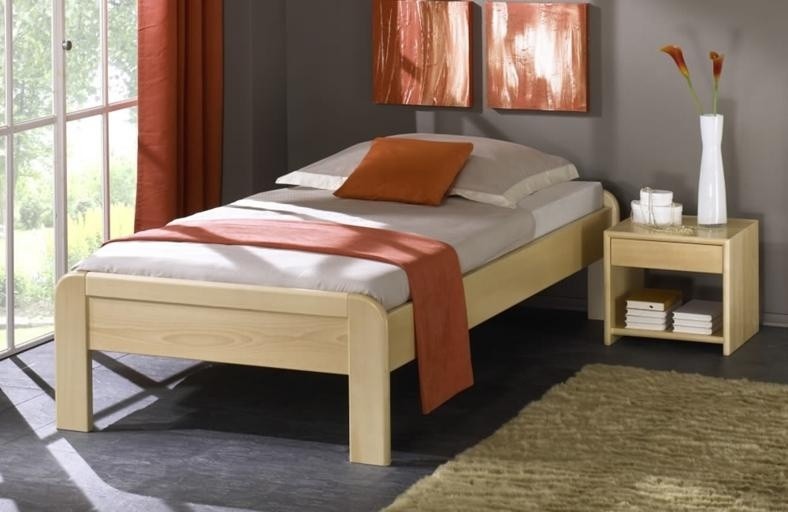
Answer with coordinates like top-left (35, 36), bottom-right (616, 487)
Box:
top-left (379, 361), bottom-right (787, 511)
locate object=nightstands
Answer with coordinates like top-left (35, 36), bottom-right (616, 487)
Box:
top-left (603, 214), bottom-right (765, 358)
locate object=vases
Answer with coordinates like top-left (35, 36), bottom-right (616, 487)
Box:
top-left (693, 110), bottom-right (730, 227)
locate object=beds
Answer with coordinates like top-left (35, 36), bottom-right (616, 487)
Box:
top-left (51, 131), bottom-right (621, 466)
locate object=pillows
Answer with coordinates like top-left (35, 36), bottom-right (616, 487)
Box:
top-left (274, 131), bottom-right (578, 210)
top-left (330, 138), bottom-right (476, 206)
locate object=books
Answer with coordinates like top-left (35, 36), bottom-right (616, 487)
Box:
top-left (622, 287), bottom-right (724, 336)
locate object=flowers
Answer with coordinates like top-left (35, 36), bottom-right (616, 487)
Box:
top-left (659, 44), bottom-right (729, 116)
